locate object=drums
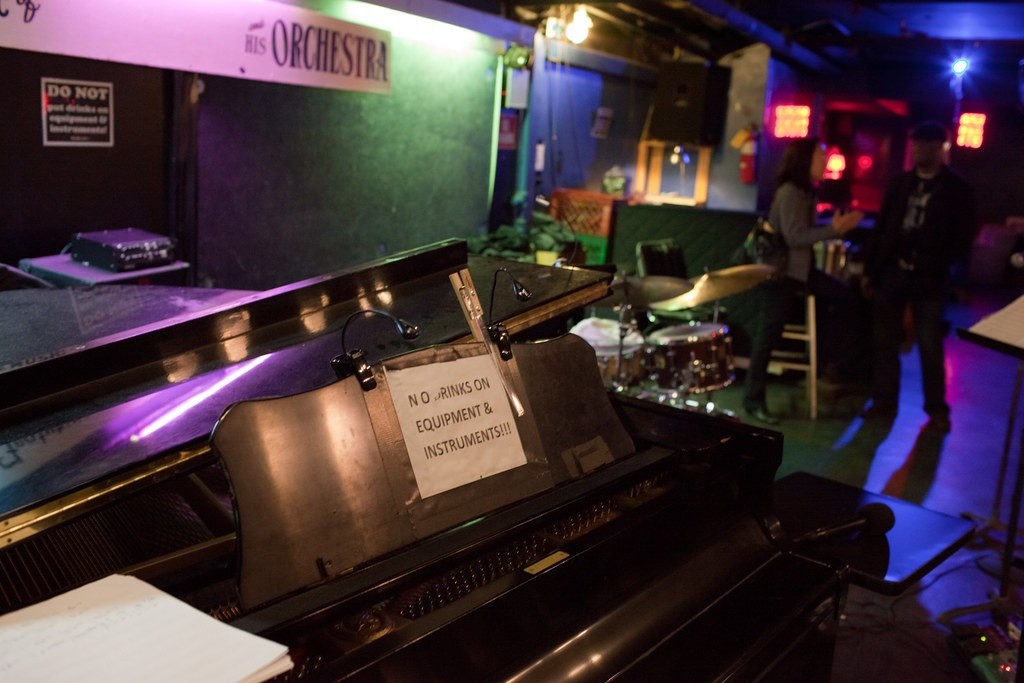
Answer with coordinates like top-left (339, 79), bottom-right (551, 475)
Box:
top-left (651, 321), bottom-right (734, 393)
top-left (571, 317), bottom-right (647, 392)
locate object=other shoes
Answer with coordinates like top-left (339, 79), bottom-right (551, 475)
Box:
top-left (745, 406), bottom-right (779, 425)
top-left (862, 401), bottom-right (897, 420)
top-left (930, 411), bottom-right (949, 431)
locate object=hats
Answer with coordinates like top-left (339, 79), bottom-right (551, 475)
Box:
top-left (913, 124), bottom-right (945, 141)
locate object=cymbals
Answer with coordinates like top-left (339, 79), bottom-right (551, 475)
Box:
top-left (586, 275), bottom-right (695, 308)
top-left (650, 264), bottom-right (779, 313)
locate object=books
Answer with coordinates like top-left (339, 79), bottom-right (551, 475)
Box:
top-left (0, 572), bottom-right (294, 683)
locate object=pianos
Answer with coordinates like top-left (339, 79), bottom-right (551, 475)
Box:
top-left (1, 238), bottom-right (898, 683)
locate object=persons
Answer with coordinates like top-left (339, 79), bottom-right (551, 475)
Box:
top-left (861, 125), bottom-right (976, 431)
top-left (744, 136), bottom-right (864, 422)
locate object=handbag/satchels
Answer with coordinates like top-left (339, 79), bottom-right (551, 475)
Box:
top-left (731, 216), bottom-right (789, 293)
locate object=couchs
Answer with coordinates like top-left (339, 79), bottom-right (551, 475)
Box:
top-left (463, 199), bottom-right (767, 347)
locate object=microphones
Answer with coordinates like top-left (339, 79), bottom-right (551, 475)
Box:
top-left (328, 307), bottom-right (422, 392)
top-left (803, 503), bottom-right (896, 543)
top-left (484, 265), bottom-right (532, 343)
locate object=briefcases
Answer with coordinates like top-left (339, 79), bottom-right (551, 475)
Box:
top-left (71, 227), bottom-right (177, 272)
